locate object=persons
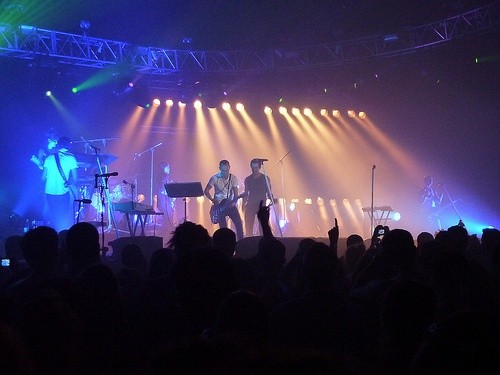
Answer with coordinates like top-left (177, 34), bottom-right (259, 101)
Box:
top-left (242, 158), bottom-right (274, 236)
top-left (204, 160), bottom-right (243, 240)
top-left (418, 176), bottom-right (444, 239)
top-left (41, 137), bottom-right (79, 232)
top-left (38, 132), bottom-right (60, 226)
top-left (156, 160), bottom-right (179, 234)
top-left (0, 217), bottom-right (500, 375)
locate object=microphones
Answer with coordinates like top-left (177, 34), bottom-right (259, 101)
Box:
top-left (74, 199), bottom-right (92, 203)
top-left (134, 153), bottom-right (137, 160)
top-left (90, 146), bottom-right (100, 150)
top-left (256, 158), bottom-right (268, 162)
top-left (96, 172), bottom-right (118, 176)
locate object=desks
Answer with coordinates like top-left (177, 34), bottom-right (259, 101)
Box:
top-left (121, 211), bottom-right (164, 237)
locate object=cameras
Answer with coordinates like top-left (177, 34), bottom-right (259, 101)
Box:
top-left (378, 229), bottom-right (384, 234)
top-left (1, 259), bottom-right (10, 266)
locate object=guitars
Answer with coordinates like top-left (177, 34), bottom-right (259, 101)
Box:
top-left (29, 153), bottom-right (79, 209)
top-left (210, 192), bottom-right (247, 224)
